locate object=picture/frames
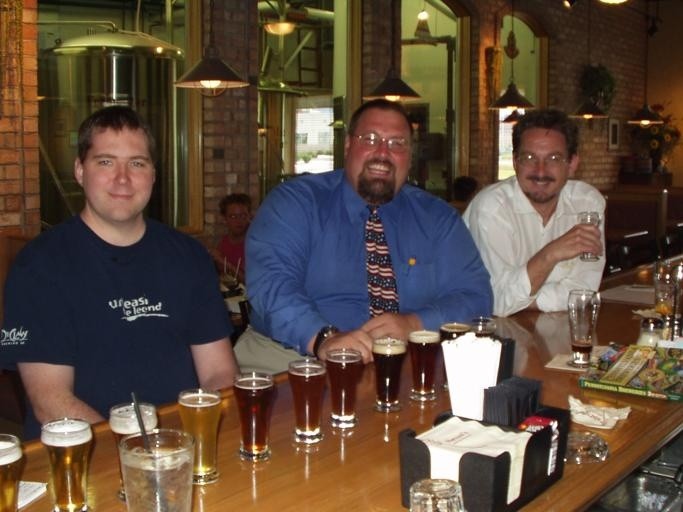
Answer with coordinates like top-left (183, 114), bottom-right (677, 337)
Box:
top-left (607, 117), bottom-right (620, 152)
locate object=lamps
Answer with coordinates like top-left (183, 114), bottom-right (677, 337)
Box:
top-left (363, 0), bottom-right (422, 100)
top-left (566, 0), bottom-right (609, 119)
top-left (171, 0), bottom-right (250, 88)
top-left (563, 0), bottom-right (663, 37)
top-left (488, 0), bottom-right (535, 110)
top-left (627, 0), bottom-right (664, 128)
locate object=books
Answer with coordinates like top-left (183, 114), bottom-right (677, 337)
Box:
top-left (578, 343), bottom-right (683, 403)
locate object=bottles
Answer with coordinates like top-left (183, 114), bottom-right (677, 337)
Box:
top-left (640, 313), bottom-right (681, 340)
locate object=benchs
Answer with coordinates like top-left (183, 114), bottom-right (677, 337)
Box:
top-left (594, 185), bottom-right (682, 270)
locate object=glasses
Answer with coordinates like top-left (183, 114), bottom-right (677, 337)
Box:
top-left (354, 134), bottom-right (407, 153)
top-left (519, 154), bottom-right (563, 165)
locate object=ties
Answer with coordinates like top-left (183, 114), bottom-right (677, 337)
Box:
top-left (365, 206), bottom-right (399, 318)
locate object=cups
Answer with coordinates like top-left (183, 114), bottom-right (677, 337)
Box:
top-left (287, 359), bottom-right (326, 444)
top-left (40, 418), bottom-right (94, 512)
top-left (371, 337), bottom-right (406, 413)
top-left (110, 402), bottom-right (159, 501)
top-left (439, 322), bottom-right (471, 390)
top-left (1, 433), bottom-right (26, 511)
top-left (324, 350), bottom-right (365, 428)
top-left (405, 329), bottom-right (441, 401)
top-left (567, 286), bottom-right (599, 366)
top-left (578, 210), bottom-right (601, 263)
top-left (117, 428), bottom-right (195, 512)
top-left (472, 316), bottom-right (498, 340)
top-left (232, 372), bottom-right (277, 463)
top-left (178, 388), bottom-right (223, 485)
top-left (653, 273), bottom-right (673, 317)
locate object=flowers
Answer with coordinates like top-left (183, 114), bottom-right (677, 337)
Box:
top-left (632, 101), bottom-right (680, 174)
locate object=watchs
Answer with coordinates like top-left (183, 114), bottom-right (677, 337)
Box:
top-left (312, 327), bottom-right (339, 357)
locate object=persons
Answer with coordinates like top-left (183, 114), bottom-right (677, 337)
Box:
top-left (210, 193), bottom-right (252, 285)
top-left (0, 105), bottom-right (241, 440)
top-left (449, 176), bottom-right (477, 213)
top-left (232, 98), bottom-right (494, 376)
top-left (460, 108), bottom-right (607, 317)
